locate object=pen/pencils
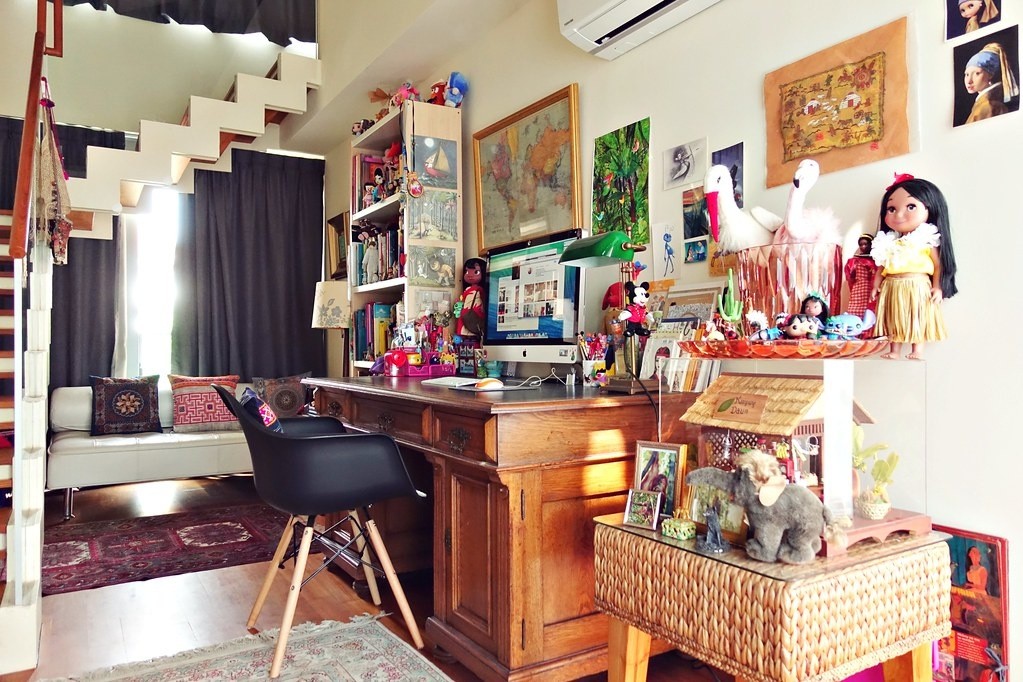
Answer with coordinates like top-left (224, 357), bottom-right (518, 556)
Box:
top-left (575, 331), bottom-right (612, 360)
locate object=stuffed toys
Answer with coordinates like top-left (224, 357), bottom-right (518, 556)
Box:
top-left (703, 159), bottom-right (863, 253)
top-left (350, 71), bottom-right (468, 210)
top-left (684, 449), bottom-right (834, 566)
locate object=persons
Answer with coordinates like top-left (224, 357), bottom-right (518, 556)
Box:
top-left (964, 43), bottom-right (1020, 125)
top-left (776, 173), bottom-right (958, 358)
top-left (963, 546), bottom-right (988, 594)
top-left (453, 257), bottom-right (487, 344)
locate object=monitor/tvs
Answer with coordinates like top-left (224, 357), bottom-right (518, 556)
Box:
top-left (481, 228), bottom-right (590, 385)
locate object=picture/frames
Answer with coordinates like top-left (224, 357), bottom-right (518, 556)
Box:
top-left (683, 479), bottom-right (751, 550)
top-left (622, 488), bottom-right (662, 531)
top-left (634, 440), bottom-right (687, 526)
top-left (472, 83), bottom-right (584, 259)
top-left (327, 210), bottom-right (350, 280)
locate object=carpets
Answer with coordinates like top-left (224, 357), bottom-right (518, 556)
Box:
top-left (34, 610), bottom-right (455, 682)
top-left (0, 499), bottom-right (323, 603)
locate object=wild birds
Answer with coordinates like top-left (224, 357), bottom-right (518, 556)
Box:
top-left (703, 159), bottom-right (842, 272)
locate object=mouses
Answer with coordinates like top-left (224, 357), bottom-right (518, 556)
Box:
top-left (475, 377), bottom-right (504, 389)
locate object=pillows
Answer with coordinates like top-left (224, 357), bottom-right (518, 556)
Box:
top-left (167, 374), bottom-right (244, 434)
top-left (239, 386), bottom-right (284, 434)
top-left (88, 374), bottom-right (164, 436)
top-left (251, 371), bottom-right (312, 419)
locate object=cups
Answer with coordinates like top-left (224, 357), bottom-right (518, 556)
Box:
top-left (582, 360), bottom-right (606, 386)
top-left (474, 349), bottom-right (484, 376)
top-left (407, 352), bottom-right (421, 365)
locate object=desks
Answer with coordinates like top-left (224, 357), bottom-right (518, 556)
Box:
top-left (592, 512), bottom-right (953, 682)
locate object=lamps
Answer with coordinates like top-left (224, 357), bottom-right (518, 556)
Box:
top-left (311, 281), bottom-right (350, 377)
top-left (558, 230), bottom-right (671, 395)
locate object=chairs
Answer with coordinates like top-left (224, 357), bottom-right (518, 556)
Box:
top-left (210, 382), bottom-right (426, 679)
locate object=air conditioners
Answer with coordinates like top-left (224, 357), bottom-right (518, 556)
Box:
top-left (556, 0), bottom-right (722, 62)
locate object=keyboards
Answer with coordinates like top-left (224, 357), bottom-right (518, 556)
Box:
top-left (421, 377), bottom-right (482, 387)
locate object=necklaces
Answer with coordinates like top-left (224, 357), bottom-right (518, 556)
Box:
top-left (973, 566), bottom-right (981, 570)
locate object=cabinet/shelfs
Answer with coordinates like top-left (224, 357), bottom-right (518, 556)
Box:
top-left (349, 100), bottom-right (464, 379)
top-left (299, 377), bottom-right (704, 682)
top-left (678, 336), bottom-right (932, 557)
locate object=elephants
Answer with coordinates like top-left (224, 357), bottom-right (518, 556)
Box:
top-left (688, 449), bottom-right (852, 565)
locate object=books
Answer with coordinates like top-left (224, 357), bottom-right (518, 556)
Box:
top-left (350, 152), bottom-right (406, 360)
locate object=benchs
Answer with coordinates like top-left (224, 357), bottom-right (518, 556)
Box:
top-left (47, 371), bottom-right (312, 520)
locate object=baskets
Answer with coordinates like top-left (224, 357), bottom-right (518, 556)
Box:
top-left (591, 522), bottom-right (952, 682)
